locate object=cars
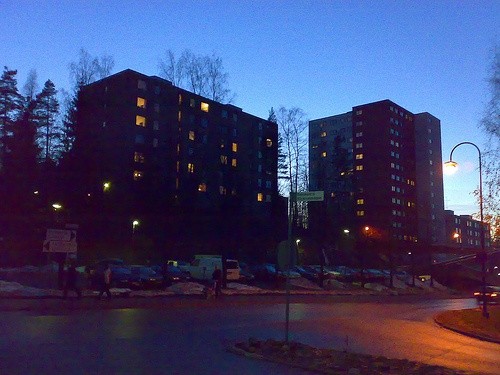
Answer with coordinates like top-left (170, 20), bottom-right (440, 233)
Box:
top-left (473, 286), bottom-right (500, 302)
top-left (83, 255), bottom-right (256, 290)
top-left (249, 264), bottom-right (432, 284)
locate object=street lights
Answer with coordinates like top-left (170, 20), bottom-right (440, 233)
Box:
top-left (443, 141), bottom-right (489, 319)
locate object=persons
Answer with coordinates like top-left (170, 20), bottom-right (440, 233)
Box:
top-left (212, 265), bottom-right (223, 295)
top-left (97, 263), bottom-right (113, 299)
top-left (65, 260), bottom-right (96, 295)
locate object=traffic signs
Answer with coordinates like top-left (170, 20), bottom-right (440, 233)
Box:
top-left (42, 230), bottom-right (77, 254)
